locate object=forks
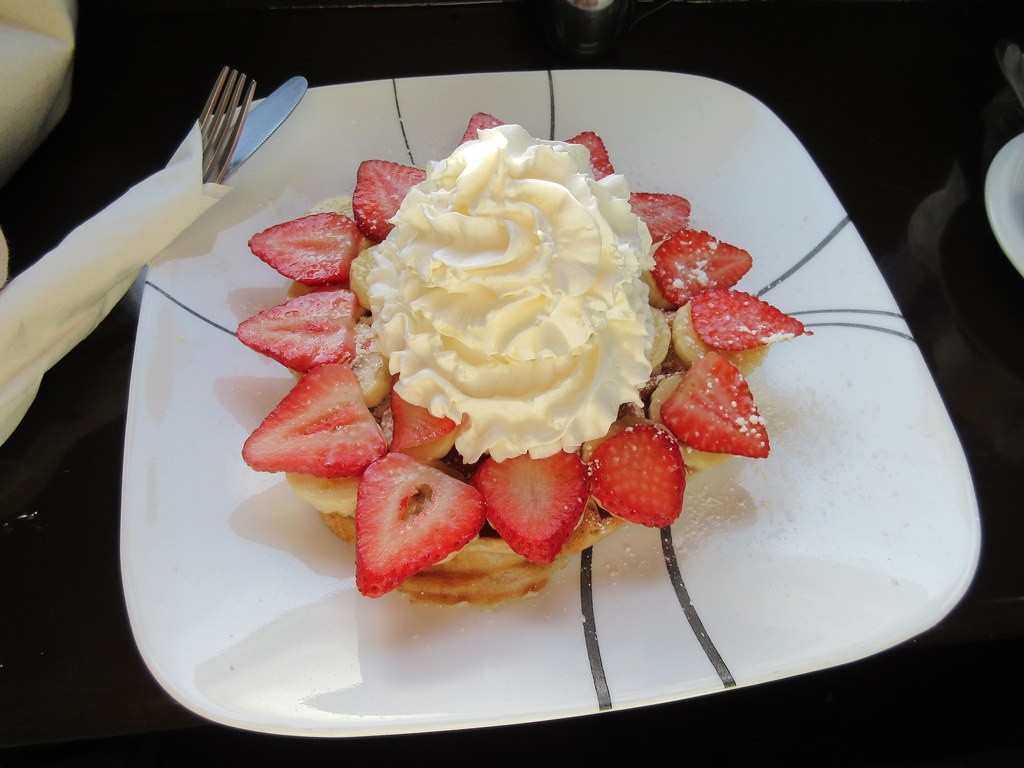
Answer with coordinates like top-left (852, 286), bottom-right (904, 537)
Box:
top-left (199, 66), bottom-right (256, 186)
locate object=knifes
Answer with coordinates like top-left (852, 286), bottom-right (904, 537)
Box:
top-left (203, 75), bottom-right (308, 185)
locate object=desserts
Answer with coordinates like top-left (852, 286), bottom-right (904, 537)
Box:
top-left (235, 112), bottom-right (808, 606)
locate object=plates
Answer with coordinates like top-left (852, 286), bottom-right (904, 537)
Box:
top-left (119, 67), bottom-right (982, 737)
top-left (984, 133), bottom-right (1024, 279)
